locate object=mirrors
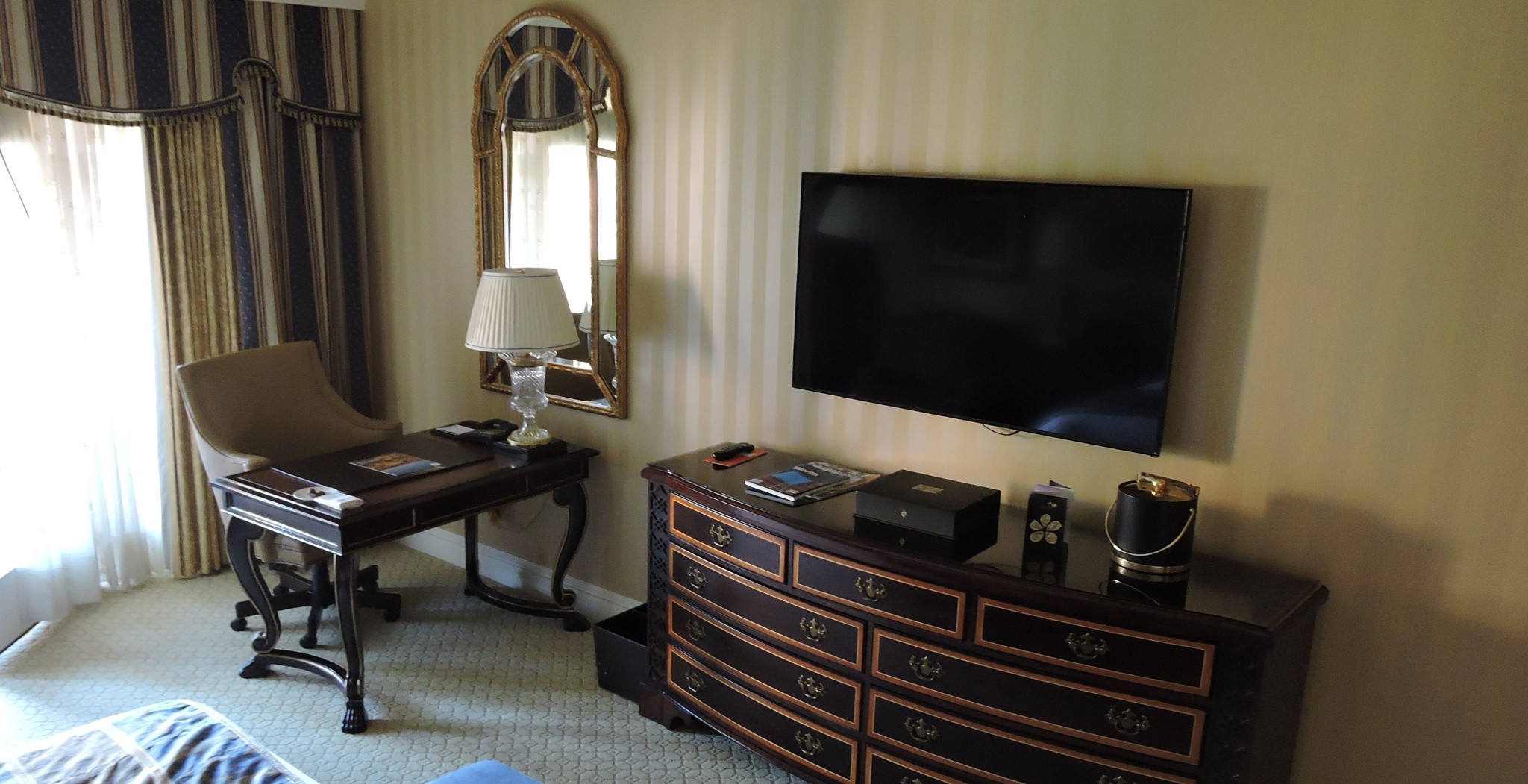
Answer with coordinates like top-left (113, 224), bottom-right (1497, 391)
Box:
top-left (469, 6), bottom-right (627, 420)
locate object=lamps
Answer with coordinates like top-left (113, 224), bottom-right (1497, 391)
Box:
top-left (464, 268), bottom-right (580, 447)
top-left (579, 259), bottom-right (616, 389)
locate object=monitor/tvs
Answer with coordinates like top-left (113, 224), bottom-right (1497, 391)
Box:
top-left (790, 171), bottom-right (1193, 459)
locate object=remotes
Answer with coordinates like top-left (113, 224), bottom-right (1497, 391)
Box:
top-left (712, 443), bottom-right (755, 460)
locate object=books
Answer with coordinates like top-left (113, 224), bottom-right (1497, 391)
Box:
top-left (347, 451), bottom-right (442, 479)
top-left (743, 462), bottom-right (880, 507)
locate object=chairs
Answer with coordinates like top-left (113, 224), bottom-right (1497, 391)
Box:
top-left (174, 341), bottom-right (403, 695)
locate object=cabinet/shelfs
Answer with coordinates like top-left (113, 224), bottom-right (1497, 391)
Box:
top-left (640, 442), bottom-right (1330, 784)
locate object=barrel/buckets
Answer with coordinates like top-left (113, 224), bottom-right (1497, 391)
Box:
top-left (1105, 479), bottom-right (1198, 584)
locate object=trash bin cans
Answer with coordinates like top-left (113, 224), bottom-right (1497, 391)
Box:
top-left (593, 601), bottom-right (647, 703)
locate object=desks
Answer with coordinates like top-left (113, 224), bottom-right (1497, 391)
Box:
top-left (209, 422), bottom-right (600, 733)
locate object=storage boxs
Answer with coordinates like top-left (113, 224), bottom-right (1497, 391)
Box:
top-left (593, 603), bottom-right (647, 705)
top-left (853, 470), bottom-right (1001, 540)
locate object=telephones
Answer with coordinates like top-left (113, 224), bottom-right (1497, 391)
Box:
top-left (466, 419), bottom-right (518, 444)
